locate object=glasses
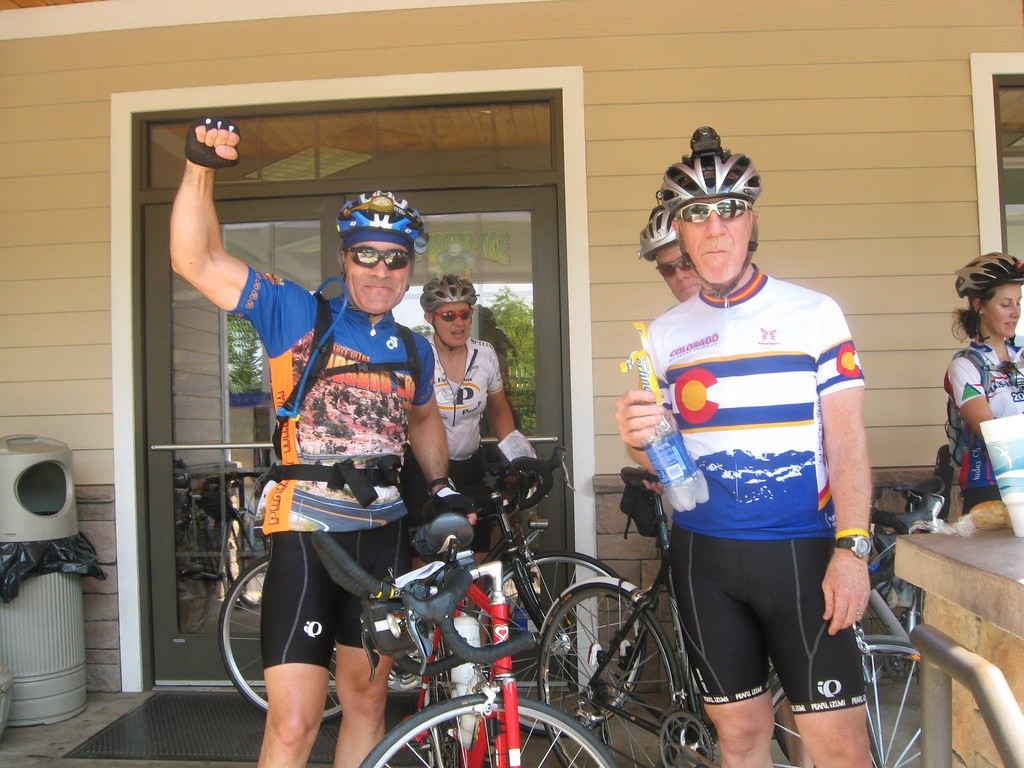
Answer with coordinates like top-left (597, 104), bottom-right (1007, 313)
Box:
top-left (346, 246), bottom-right (412, 270)
top-left (434, 308), bottom-right (474, 322)
top-left (655, 254), bottom-right (691, 278)
top-left (675, 198), bottom-right (750, 223)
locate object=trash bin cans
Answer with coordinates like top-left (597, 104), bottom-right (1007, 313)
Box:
top-left (0, 435), bottom-right (88, 728)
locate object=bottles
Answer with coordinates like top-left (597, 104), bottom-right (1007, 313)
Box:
top-left (642, 407), bottom-right (708, 512)
top-left (450, 610), bottom-right (489, 750)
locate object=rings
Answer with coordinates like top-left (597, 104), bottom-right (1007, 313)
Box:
top-left (856, 612), bottom-right (864, 616)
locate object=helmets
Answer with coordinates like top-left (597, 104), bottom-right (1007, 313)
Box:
top-left (640, 206), bottom-right (678, 261)
top-left (956, 251), bottom-right (1024, 298)
top-left (661, 150), bottom-right (763, 214)
top-left (420, 274), bottom-right (477, 312)
top-left (335, 190), bottom-right (430, 254)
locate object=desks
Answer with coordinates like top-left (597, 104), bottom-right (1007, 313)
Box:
top-left (894, 527), bottom-right (1024, 768)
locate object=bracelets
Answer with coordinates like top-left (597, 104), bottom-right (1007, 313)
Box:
top-left (835, 528), bottom-right (870, 540)
top-left (630, 447), bottom-right (650, 451)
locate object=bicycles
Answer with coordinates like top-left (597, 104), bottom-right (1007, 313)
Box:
top-left (537, 466), bottom-right (922, 768)
top-left (218, 445), bottom-right (647, 739)
top-left (858, 473), bottom-right (949, 637)
top-left (173, 457), bottom-right (273, 634)
top-left (304, 511), bottom-right (620, 767)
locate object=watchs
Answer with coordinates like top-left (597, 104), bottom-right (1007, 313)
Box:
top-left (427, 476), bottom-right (456, 495)
top-left (833, 535), bottom-right (872, 558)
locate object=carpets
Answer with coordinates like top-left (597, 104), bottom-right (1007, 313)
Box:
top-left (59, 689), bottom-right (493, 768)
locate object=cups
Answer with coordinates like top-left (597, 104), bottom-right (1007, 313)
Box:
top-left (979, 414), bottom-right (1024, 537)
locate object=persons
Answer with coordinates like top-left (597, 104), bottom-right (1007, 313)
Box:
top-left (637, 205), bottom-right (814, 768)
top-left (388, 273), bottom-right (547, 666)
top-left (943, 251), bottom-right (1024, 516)
top-left (169, 115), bottom-right (476, 768)
top-left (615, 126), bottom-right (872, 768)
